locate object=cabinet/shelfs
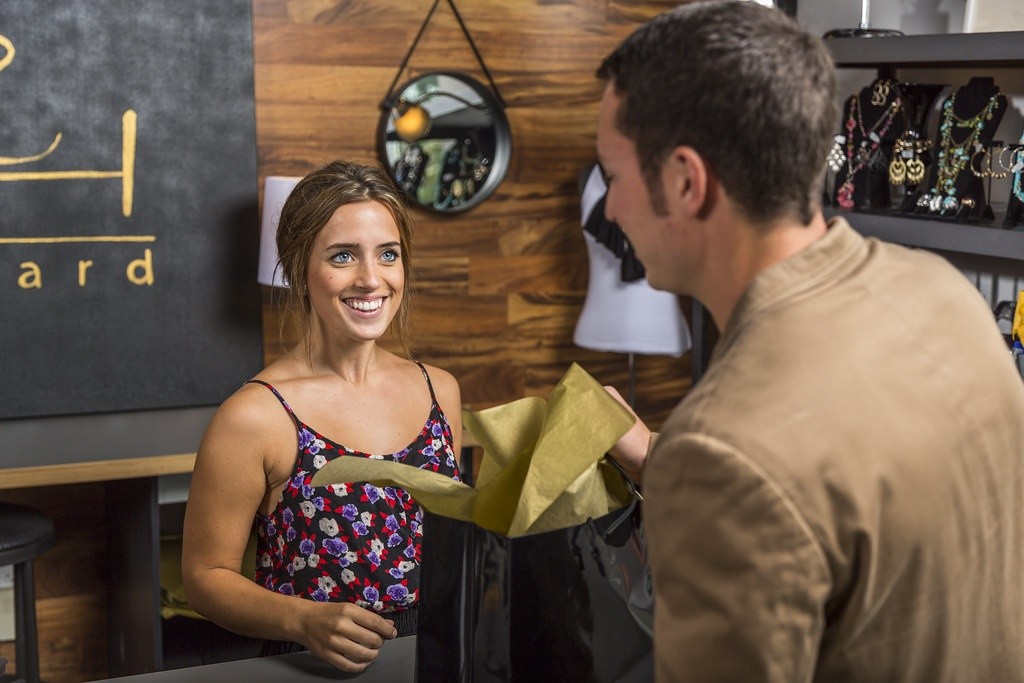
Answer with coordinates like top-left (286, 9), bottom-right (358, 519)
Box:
top-left (811, 24), bottom-right (1024, 353)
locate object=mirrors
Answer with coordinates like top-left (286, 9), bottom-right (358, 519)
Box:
top-left (374, 0), bottom-right (513, 215)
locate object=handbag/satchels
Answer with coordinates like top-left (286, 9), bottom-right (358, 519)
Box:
top-left (414, 446), bottom-right (508, 682)
top-left (507, 447), bottom-right (655, 683)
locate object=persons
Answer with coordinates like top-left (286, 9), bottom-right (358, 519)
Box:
top-left (590, 0), bottom-right (1023, 683)
top-left (178, 160), bottom-right (463, 675)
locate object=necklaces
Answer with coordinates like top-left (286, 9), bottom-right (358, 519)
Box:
top-left (831, 79), bottom-right (1023, 220)
